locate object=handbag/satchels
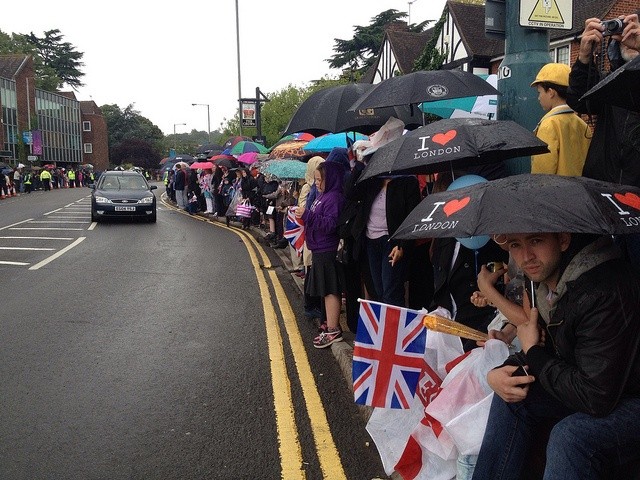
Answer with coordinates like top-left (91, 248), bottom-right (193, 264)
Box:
top-left (236, 199), bottom-right (251, 217)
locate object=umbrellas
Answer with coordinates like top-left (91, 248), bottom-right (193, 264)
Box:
top-left (353, 117), bottom-right (552, 187)
top-left (281, 82), bottom-right (422, 134)
top-left (158, 130), bottom-right (369, 180)
top-left (343, 70), bottom-right (505, 113)
top-left (418, 74), bottom-right (501, 114)
top-left (385, 172), bottom-right (639, 242)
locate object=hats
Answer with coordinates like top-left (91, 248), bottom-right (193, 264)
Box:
top-left (530, 64), bottom-right (571, 87)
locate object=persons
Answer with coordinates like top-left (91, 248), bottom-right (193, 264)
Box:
top-left (530, 62), bottom-right (592, 176)
top-left (344, 151), bottom-right (422, 308)
top-left (0, 163), bottom-right (98, 200)
top-left (297, 155), bottom-right (324, 318)
top-left (160, 164), bottom-right (296, 249)
top-left (326, 147), bottom-right (349, 177)
top-left (304, 161), bottom-right (343, 348)
top-left (472, 233), bottom-right (639, 480)
top-left (568, 12), bottom-right (639, 182)
top-left (410, 176), bottom-right (506, 355)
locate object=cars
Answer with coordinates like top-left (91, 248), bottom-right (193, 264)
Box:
top-left (87, 169), bottom-right (157, 223)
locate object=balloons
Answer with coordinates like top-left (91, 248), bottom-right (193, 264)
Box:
top-left (445, 174), bottom-right (494, 289)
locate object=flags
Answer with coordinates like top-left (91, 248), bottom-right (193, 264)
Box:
top-left (353, 297), bottom-right (427, 410)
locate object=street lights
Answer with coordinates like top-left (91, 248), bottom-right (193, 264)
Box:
top-left (174, 123), bottom-right (186, 149)
top-left (192, 104), bottom-right (211, 144)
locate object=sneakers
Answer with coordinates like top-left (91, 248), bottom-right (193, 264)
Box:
top-left (313, 328), bottom-right (343, 349)
